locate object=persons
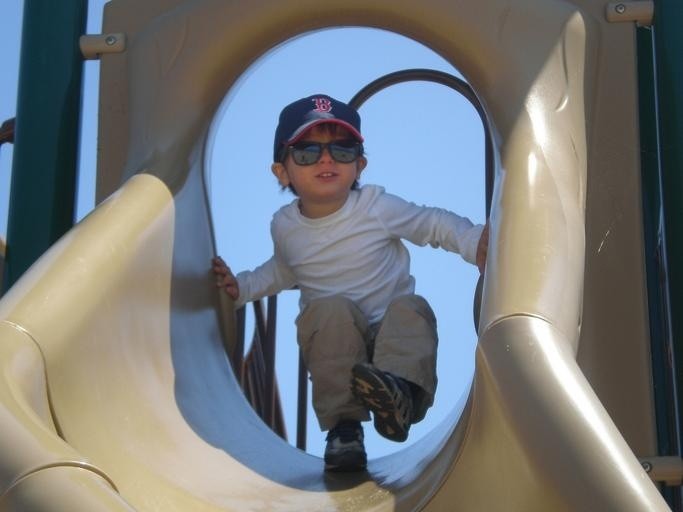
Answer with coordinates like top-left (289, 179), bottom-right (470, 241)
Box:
top-left (211, 93), bottom-right (490, 477)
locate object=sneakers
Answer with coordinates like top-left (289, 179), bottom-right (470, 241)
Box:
top-left (325, 422), bottom-right (367, 471)
top-left (351, 363), bottom-right (414, 442)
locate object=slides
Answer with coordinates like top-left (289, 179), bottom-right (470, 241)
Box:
top-left (0, 158), bottom-right (673, 512)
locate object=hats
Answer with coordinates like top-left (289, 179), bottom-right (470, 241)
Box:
top-left (273, 94), bottom-right (363, 161)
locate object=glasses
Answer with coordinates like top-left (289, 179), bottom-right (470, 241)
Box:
top-left (281, 140), bottom-right (361, 165)
